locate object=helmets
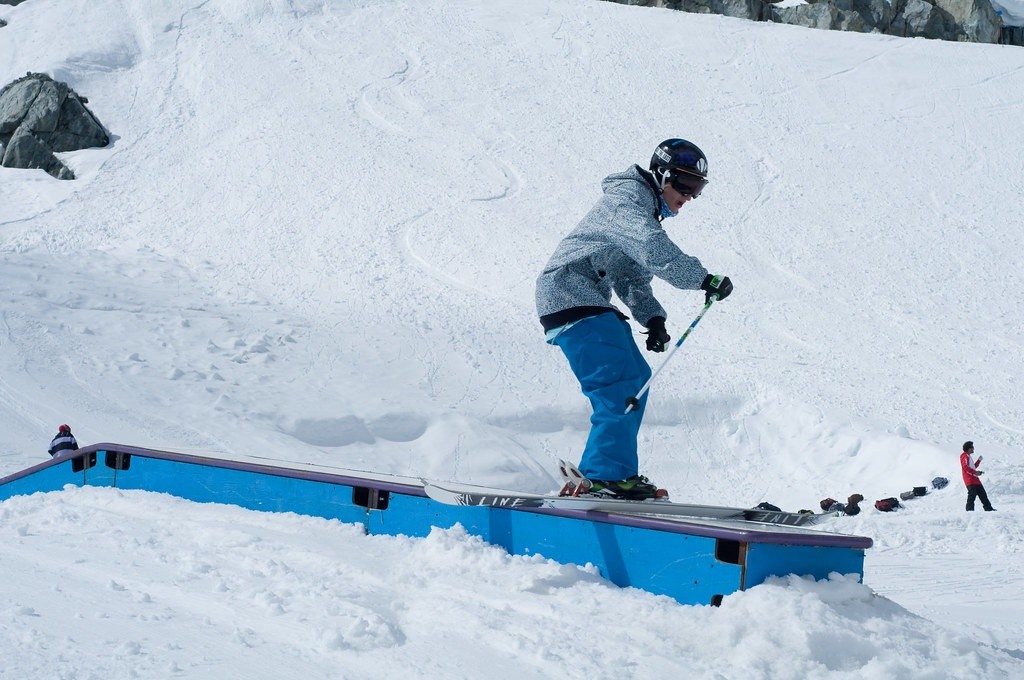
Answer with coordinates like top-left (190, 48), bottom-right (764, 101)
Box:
top-left (649, 138), bottom-right (708, 177)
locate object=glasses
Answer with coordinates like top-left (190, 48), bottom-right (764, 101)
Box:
top-left (665, 167), bottom-right (709, 199)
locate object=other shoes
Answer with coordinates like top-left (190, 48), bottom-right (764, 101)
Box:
top-left (991, 509), bottom-right (996, 511)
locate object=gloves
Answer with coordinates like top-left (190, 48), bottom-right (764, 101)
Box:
top-left (640, 316), bottom-right (671, 352)
top-left (701, 274), bottom-right (733, 305)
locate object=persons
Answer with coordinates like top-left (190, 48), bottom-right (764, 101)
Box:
top-left (534, 138), bottom-right (734, 501)
top-left (48, 424), bottom-right (79, 459)
top-left (960, 441), bottom-right (997, 512)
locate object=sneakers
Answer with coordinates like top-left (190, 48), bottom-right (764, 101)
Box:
top-left (577, 459), bottom-right (668, 501)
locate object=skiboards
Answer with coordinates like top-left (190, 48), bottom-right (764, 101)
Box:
top-left (422, 481), bottom-right (841, 527)
